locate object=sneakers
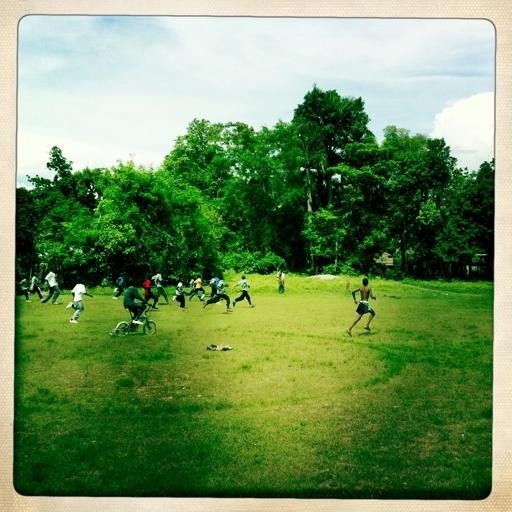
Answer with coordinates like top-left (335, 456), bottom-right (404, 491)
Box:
top-left (172, 295), bottom-right (185, 311)
top-left (226, 301), bottom-right (236, 312)
top-left (364, 326), bottom-right (372, 331)
top-left (112, 289), bottom-right (119, 300)
top-left (140, 301), bottom-right (158, 312)
top-left (346, 329), bottom-right (352, 337)
top-left (132, 319), bottom-right (143, 325)
top-left (66, 302), bottom-right (73, 310)
top-left (25, 297), bottom-right (61, 306)
top-left (200, 294), bottom-right (225, 304)
top-left (249, 303), bottom-right (256, 307)
top-left (70, 319), bottom-right (78, 324)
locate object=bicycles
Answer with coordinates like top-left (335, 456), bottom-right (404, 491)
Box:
top-left (113, 303), bottom-right (159, 338)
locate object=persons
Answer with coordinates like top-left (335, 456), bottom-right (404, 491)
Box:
top-left (18, 277), bottom-right (30, 303)
top-left (66, 280), bottom-right (93, 324)
top-left (123, 282), bottom-right (147, 325)
top-left (275, 267), bottom-right (285, 294)
top-left (345, 278), bottom-right (377, 337)
top-left (112, 272), bottom-right (230, 310)
top-left (28, 273), bottom-right (43, 299)
top-left (40, 269), bottom-right (60, 305)
top-left (232, 275), bottom-right (255, 308)
